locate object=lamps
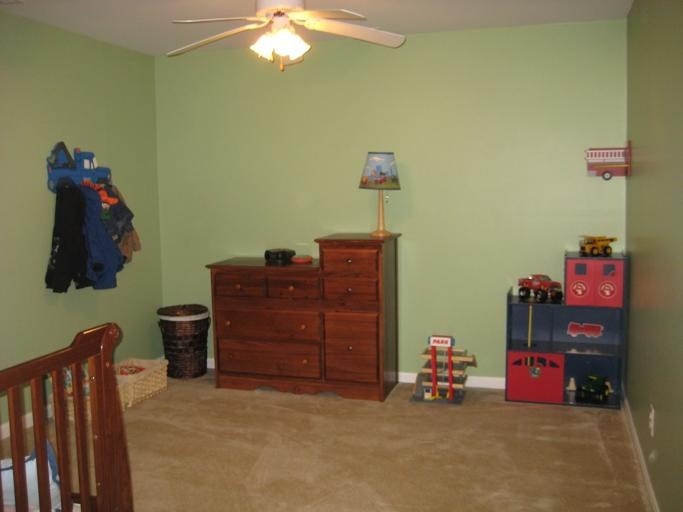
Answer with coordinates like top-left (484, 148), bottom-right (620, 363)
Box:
top-left (358, 150), bottom-right (401, 239)
top-left (248, 17), bottom-right (311, 74)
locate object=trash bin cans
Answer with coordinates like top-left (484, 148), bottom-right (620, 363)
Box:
top-left (156, 304), bottom-right (211, 379)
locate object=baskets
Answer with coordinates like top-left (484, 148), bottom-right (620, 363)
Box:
top-left (51, 377), bottom-right (129, 427)
top-left (107, 355), bottom-right (170, 414)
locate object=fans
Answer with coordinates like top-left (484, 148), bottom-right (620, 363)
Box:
top-left (162, 2), bottom-right (409, 59)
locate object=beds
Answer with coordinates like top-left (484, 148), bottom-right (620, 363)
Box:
top-left (0, 317), bottom-right (143, 512)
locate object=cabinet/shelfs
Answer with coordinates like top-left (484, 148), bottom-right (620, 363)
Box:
top-left (204, 230), bottom-right (402, 403)
top-left (500, 248), bottom-right (633, 412)
